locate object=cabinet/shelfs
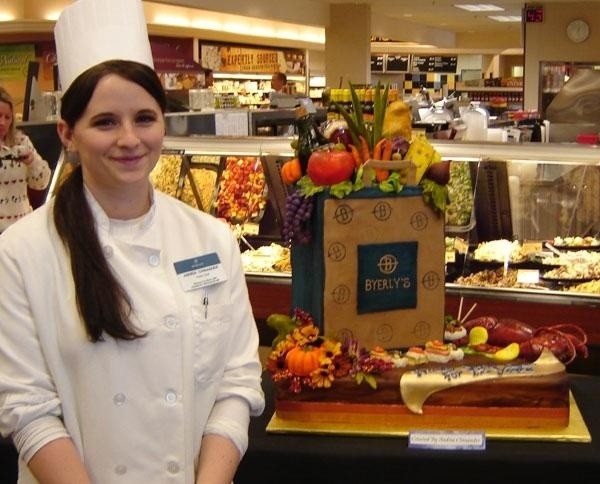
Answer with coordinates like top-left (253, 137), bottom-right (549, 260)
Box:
top-left (449, 84), bottom-right (524, 117)
top-left (44, 130), bottom-right (600, 373)
top-left (209, 74), bottom-right (305, 108)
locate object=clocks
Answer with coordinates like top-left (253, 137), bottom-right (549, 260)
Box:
top-left (566, 18), bottom-right (592, 45)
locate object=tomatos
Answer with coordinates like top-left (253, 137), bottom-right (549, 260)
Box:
top-left (308, 148), bottom-right (353, 186)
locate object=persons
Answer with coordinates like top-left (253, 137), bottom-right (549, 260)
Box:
top-left (260, 72), bottom-right (295, 136)
top-left (0, 0), bottom-right (266, 484)
top-left (202, 68), bottom-right (213, 89)
top-left (0, 85), bottom-right (51, 236)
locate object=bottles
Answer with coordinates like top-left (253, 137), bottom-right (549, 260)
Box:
top-left (420, 103), bottom-right (457, 140)
top-left (478, 71), bottom-right (501, 86)
top-left (462, 101), bottom-right (488, 140)
top-left (326, 87), bottom-right (398, 125)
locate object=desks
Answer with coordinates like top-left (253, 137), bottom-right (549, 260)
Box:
top-left (243, 346), bottom-right (600, 483)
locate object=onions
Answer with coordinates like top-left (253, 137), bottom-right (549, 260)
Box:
top-left (427, 159), bottom-right (452, 185)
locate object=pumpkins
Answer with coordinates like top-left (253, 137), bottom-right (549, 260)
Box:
top-left (280, 158), bottom-right (302, 186)
top-left (283, 344), bottom-right (325, 376)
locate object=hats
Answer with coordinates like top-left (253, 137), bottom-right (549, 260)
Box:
top-left (52, 0), bottom-right (156, 101)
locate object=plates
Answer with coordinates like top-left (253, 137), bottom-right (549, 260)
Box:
top-left (472, 243), bottom-right (600, 282)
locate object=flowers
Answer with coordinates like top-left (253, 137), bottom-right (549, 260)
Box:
top-left (266, 321), bottom-right (363, 395)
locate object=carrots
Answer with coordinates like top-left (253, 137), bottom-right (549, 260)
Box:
top-left (348, 134), bottom-right (393, 181)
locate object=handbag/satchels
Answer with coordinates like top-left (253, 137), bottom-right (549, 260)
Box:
top-left (288, 182), bottom-right (450, 354)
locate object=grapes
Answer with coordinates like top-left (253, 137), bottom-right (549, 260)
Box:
top-left (281, 189), bottom-right (315, 247)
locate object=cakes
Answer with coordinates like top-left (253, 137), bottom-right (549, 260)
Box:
top-left (370, 320), bottom-right (467, 369)
